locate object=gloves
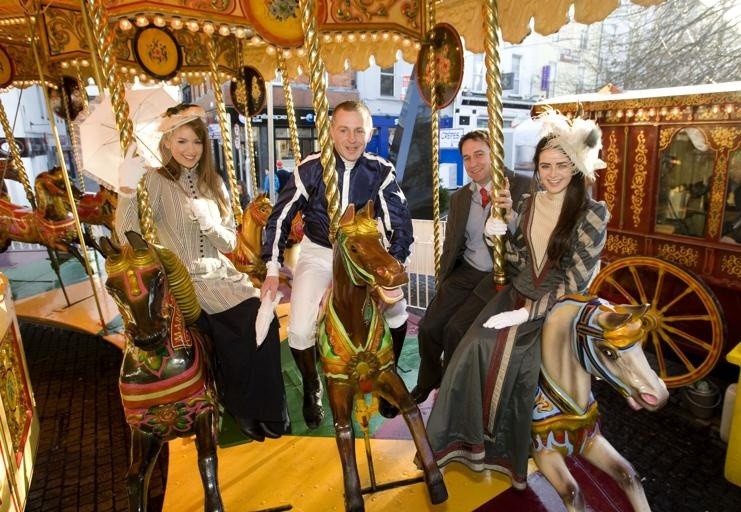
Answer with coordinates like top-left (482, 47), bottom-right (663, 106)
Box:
top-left (190, 198), bottom-right (222, 231)
top-left (253, 289), bottom-right (283, 347)
top-left (485, 217), bottom-right (508, 236)
top-left (482, 307), bottom-right (530, 330)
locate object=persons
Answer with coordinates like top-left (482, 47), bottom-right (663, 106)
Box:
top-left (408, 129), bottom-right (545, 404)
top-left (413, 100), bottom-right (612, 490)
top-left (275, 161), bottom-right (291, 191)
top-left (115, 100), bottom-right (293, 442)
top-left (264, 168), bottom-right (279, 205)
top-left (260, 100), bottom-right (415, 430)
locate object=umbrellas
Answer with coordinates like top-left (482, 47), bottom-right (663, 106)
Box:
top-left (69, 81), bottom-right (185, 199)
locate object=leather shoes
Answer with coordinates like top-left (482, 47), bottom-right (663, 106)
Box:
top-left (233, 413), bottom-right (266, 443)
top-left (411, 383), bottom-right (441, 404)
top-left (256, 421), bottom-right (283, 439)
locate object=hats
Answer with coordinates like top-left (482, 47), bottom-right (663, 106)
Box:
top-left (156, 103), bottom-right (205, 133)
top-left (556, 119), bottom-right (602, 177)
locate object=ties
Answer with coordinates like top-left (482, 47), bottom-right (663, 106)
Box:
top-left (479, 187), bottom-right (491, 209)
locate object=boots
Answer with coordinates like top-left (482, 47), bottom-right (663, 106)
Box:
top-left (378, 320), bottom-right (408, 419)
top-left (288, 346), bottom-right (324, 430)
